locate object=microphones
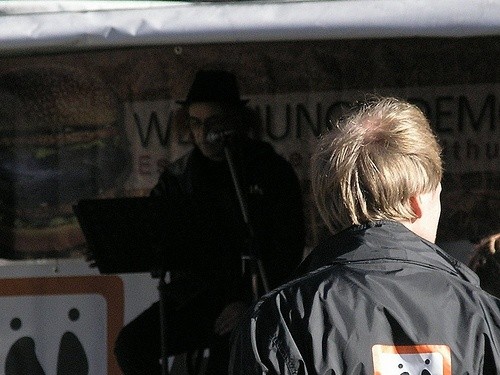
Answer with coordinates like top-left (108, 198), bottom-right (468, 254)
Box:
top-left (206, 129), bottom-right (236, 144)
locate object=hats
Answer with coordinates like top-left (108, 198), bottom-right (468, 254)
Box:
top-left (175, 70), bottom-right (250, 106)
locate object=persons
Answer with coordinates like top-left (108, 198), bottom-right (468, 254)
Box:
top-left (223, 96), bottom-right (500, 375)
top-left (112, 64), bottom-right (308, 375)
top-left (468, 233), bottom-right (500, 301)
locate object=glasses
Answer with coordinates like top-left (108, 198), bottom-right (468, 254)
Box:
top-left (184, 115), bottom-right (239, 129)
